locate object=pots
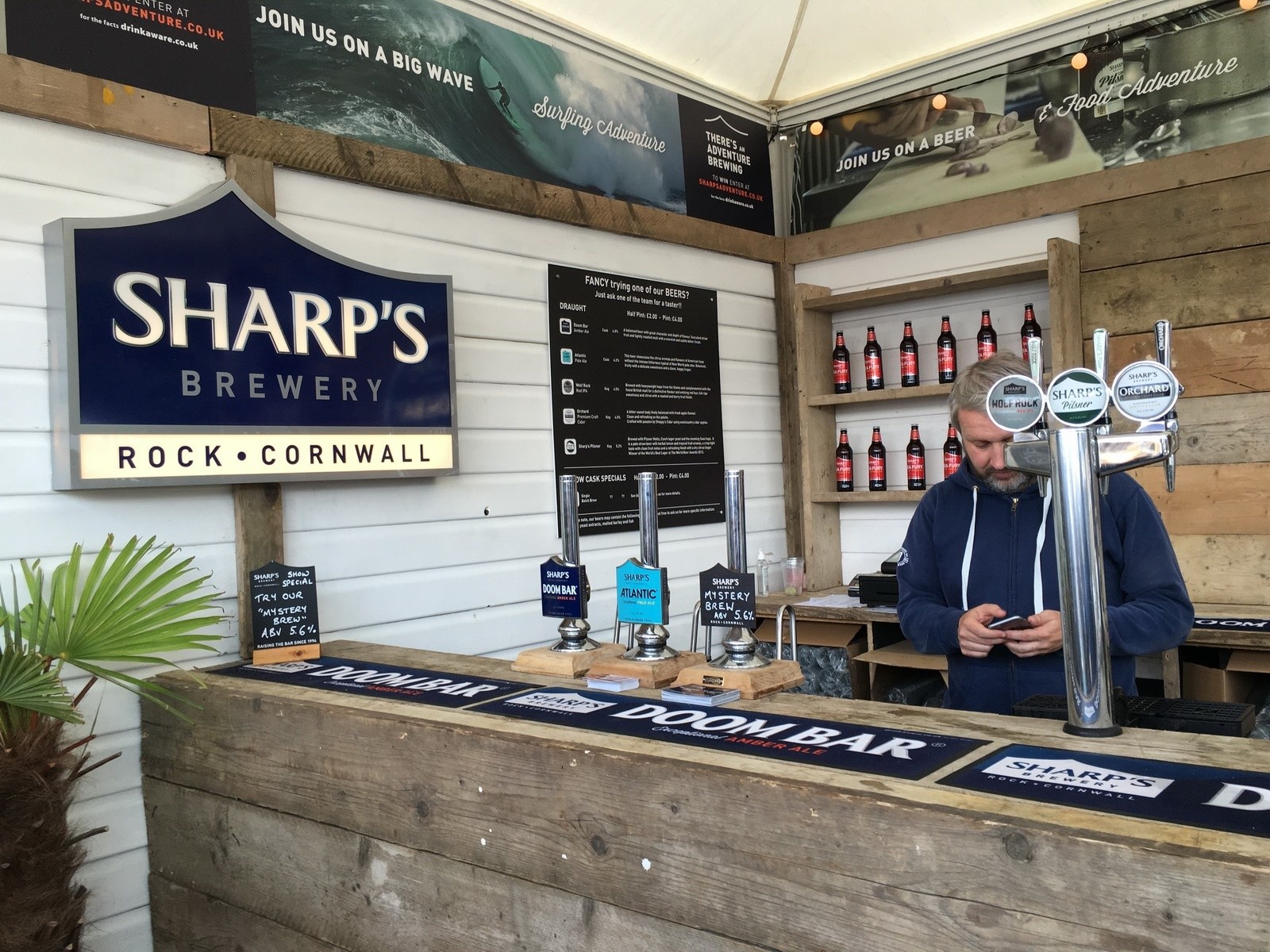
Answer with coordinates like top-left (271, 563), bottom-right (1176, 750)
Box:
top-left (1047, 3), bottom-right (1270, 108)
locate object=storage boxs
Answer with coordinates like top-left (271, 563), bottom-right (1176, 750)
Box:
top-left (754, 617), bottom-right (869, 699)
top-left (1178, 651), bottom-right (1270, 704)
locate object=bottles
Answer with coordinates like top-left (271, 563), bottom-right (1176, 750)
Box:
top-left (868, 426), bottom-right (887, 491)
top-left (906, 424), bottom-right (926, 491)
top-left (1078, 29), bottom-right (1124, 136)
top-left (863, 325), bottom-right (885, 391)
top-left (976, 309), bottom-right (997, 361)
top-left (899, 321), bottom-right (920, 387)
top-left (1020, 303), bottom-right (1045, 373)
top-left (943, 421), bottom-right (963, 481)
top-left (937, 315), bottom-right (958, 384)
top-left (835, 428), bottom-right (854, 492)
top-left (831, 330), bottom-right (853, 394)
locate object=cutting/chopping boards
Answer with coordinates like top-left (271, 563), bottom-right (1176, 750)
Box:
top-left (829, 108), bottom-right (1106, 230)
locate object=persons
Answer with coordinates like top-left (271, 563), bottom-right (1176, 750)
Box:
top-left (870, 93), bottom-right (986, 139)
top-left (897, 352), bottom-right (1194, 714)
top-left (485, 81), bottom-right (512, 116)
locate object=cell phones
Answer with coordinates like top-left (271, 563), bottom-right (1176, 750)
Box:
top-left (988, 615), bottom-right (1036, 641)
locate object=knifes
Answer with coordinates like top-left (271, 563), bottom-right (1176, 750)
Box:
top-left (904, 106), bottom-right (1026, 152)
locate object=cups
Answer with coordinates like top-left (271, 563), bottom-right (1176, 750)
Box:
top-left (780, 557), bottom-right (805, 596)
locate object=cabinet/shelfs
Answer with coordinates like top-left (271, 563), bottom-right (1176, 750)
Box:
top-left (791, 236), bottom-right (1082, 589)
